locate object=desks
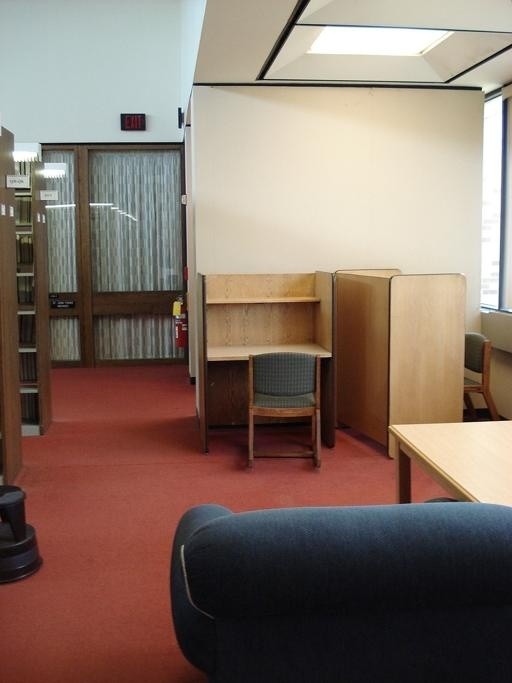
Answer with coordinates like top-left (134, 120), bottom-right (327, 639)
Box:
top-left (198, 342), bottom-right (337, 451)
top-left (388, 418), bottom-right (512, 516)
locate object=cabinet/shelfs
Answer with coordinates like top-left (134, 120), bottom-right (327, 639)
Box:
top-left (11, 161), bottom-right (52, 435)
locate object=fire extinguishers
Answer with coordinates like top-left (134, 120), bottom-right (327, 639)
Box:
top-left (171, 293), bottom-right (188, 349)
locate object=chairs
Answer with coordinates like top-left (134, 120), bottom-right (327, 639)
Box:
top-left (461, 329), bottom-right (498, 422)
top-left (245, 352), bottom-right (324, 470)
top-left (168, 502), bottom-right (511, 681)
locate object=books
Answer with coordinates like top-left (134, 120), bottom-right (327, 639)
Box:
top-left (15, 157), bottom-right (39, 432)
